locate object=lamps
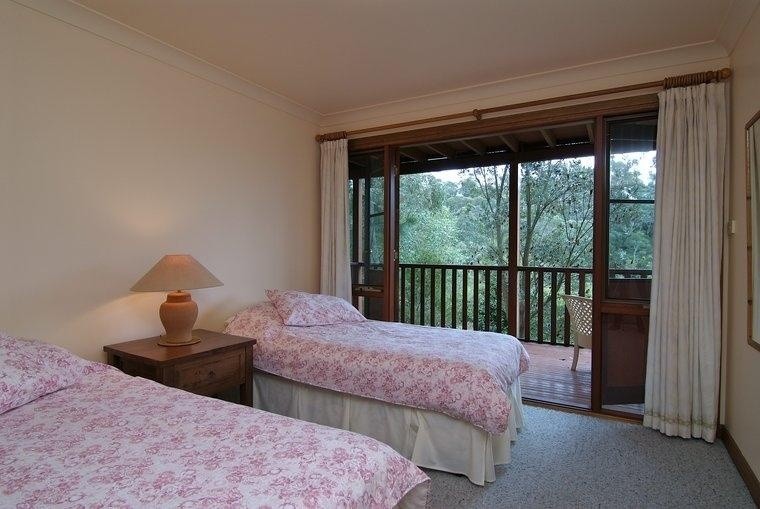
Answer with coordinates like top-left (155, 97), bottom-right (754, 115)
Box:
top-left (130, 254), bottom-right (224, 347)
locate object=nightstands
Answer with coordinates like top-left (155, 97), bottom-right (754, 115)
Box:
top-left (103, 328), bottom-right (257, 407)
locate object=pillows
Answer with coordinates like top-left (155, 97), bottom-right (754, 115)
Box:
top-left (1, 336), bottom-right (102, 414)
top-left (264, 289), bottom-right (368, 326)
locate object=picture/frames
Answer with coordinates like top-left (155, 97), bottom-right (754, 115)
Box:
top-left (744, 109), bottom-right (760, 353)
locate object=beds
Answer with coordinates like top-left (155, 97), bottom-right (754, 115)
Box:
top-left (0, 336), bottom-right (432, 509)
top-left (223, 301), bottom-right (531, 487)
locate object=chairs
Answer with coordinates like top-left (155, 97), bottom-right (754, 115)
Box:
top-left (560, 294), bottom-right (593, 371)
